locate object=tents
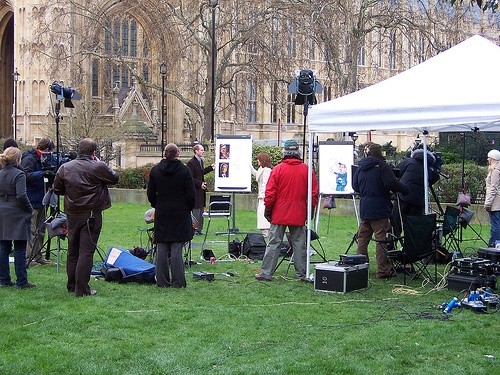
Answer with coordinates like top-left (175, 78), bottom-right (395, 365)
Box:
top-left (306, 34), bottom-right (500, 278)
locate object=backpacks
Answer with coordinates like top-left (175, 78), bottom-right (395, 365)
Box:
top-left (243, 233), bottom-right (266, 259)
top-left (230, 238), bottom-right (241, 259)
top-left (280, 241), bottom-right (293, 257)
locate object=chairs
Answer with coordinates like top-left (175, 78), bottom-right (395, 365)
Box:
top-left (139, 228), bottom-right (192, 269)
top-left (386, 205), bottom-right (464, 285)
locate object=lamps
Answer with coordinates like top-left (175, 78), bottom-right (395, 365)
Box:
top-left (287, 69), bottom-right (324, 108)
top-left (50, 84), bottom-right (83, 110)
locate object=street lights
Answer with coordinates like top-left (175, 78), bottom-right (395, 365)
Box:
top-left (159, 61), bottom-right (168, 158)
top-left (207, 0), bottom-right (220, 143)
top-left (12, 68), bottom-right (21, 140)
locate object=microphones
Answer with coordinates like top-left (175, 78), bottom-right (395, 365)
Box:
top-left (61, 151), bottom-right (77, 160)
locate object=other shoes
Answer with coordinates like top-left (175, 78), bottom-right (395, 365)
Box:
top-left (16, 283), bottom-right (36, 290)
top-left (385, 272), bottom-right (397, 278)
top-left (74, 290), bottom-right (97, 297)
top-left (0, 282), bottom-right (15, 287)
top-left (27, 258), bottom-right (52, 268)
top-left (255, 273), bottom-right (273, 282)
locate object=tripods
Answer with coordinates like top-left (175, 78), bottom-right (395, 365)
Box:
top-left (442, 132), bottom-right (489, 247)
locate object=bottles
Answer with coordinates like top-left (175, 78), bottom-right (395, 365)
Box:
top-left (452, 251), bottom-right (457, 260)
top-left (443, 297), bottom-right (462, 314)
top-left (491, 240), bottom-right (500, 250)
top-left (469, 288), bottom-right (490, 302)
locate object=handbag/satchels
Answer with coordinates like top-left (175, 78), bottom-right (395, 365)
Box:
top-left (486, 207), bottom-right (491, 212)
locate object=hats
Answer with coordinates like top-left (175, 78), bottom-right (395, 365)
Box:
top-left (487, 150), bottom-right (500, 161)
top-left (285, 139), bottom-right (299, 150)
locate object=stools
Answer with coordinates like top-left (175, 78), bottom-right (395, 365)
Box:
top-left (202, 202), bottom-right (232, 255)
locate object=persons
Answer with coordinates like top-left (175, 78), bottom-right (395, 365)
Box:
top-left (364, 142), bottom-right (440, 264)
top-left (351, 144), bottom-right (407, 279)
top-left (187, 145), bottom-right (216, 236)
top-left (0, 138), bottom-right (36, 288)
top-left (21, 138), bottom-right (55, 267)
top-left (146, 143), bottom-right (193, 288)
top-left (255, 140), bottom-right (319, 282)
top-left (54, 138), bottom-right (119, 296)
top-left (220, 164), bottom-right (228, 177)
top-left (251, 152), bottom-right (273, 238)
top-left (484, 150), bottom-right (500, 248)
top-left (220, 145), bottom-right (229, 159)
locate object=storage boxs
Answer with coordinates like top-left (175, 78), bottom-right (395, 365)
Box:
top-left (313, 261), bottom-right (370, 295)
top-left (477, 247), bottom-right (500, 275)
top-left (446, 275), bottom-right (497, 292)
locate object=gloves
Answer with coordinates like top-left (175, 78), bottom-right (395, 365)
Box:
top-left (45, 169), bottom-right (56, 176)
top-left (306, 210), bottom-right (314, 220)
top-left (263, 209), bottom-right (271, 222)
